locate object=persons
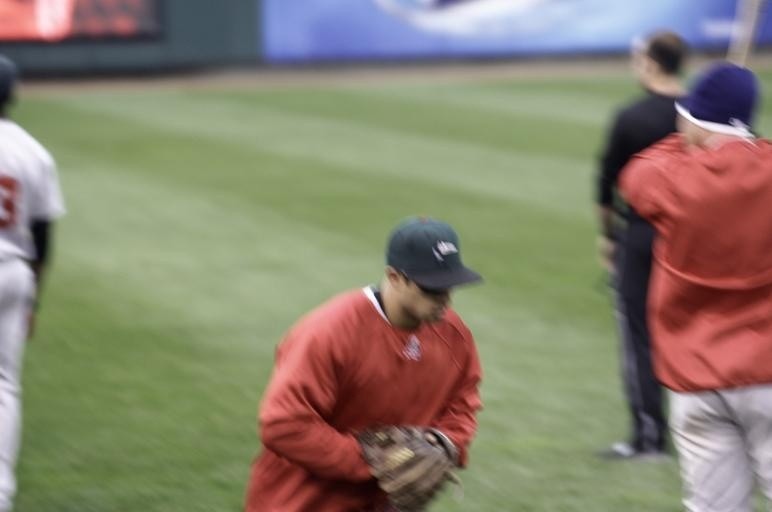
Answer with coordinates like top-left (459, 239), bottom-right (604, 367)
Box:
top-left (594, 26), bottom-right (691, 460)
top-left (611, 58), bottom-right (770, 510)
top-left (243, 219), bottom-right (485, 511)
top-left (1, 53), bottom-right (66, 511)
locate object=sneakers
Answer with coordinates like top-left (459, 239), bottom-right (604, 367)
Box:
top-left (612, 435), bottom-right (672, 465)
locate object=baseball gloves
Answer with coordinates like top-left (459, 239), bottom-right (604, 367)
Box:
top-left (352, 425), bottom-right (460, 511)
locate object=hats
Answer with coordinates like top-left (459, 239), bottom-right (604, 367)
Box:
top-left (647, 32), bottom-right (687, 73)
top-left (386, 219), bottom-right (484, 291)
top-left (0, 54), bottom-right (18, 101)
top-left (673, 61), bottom-right (761, 140)
